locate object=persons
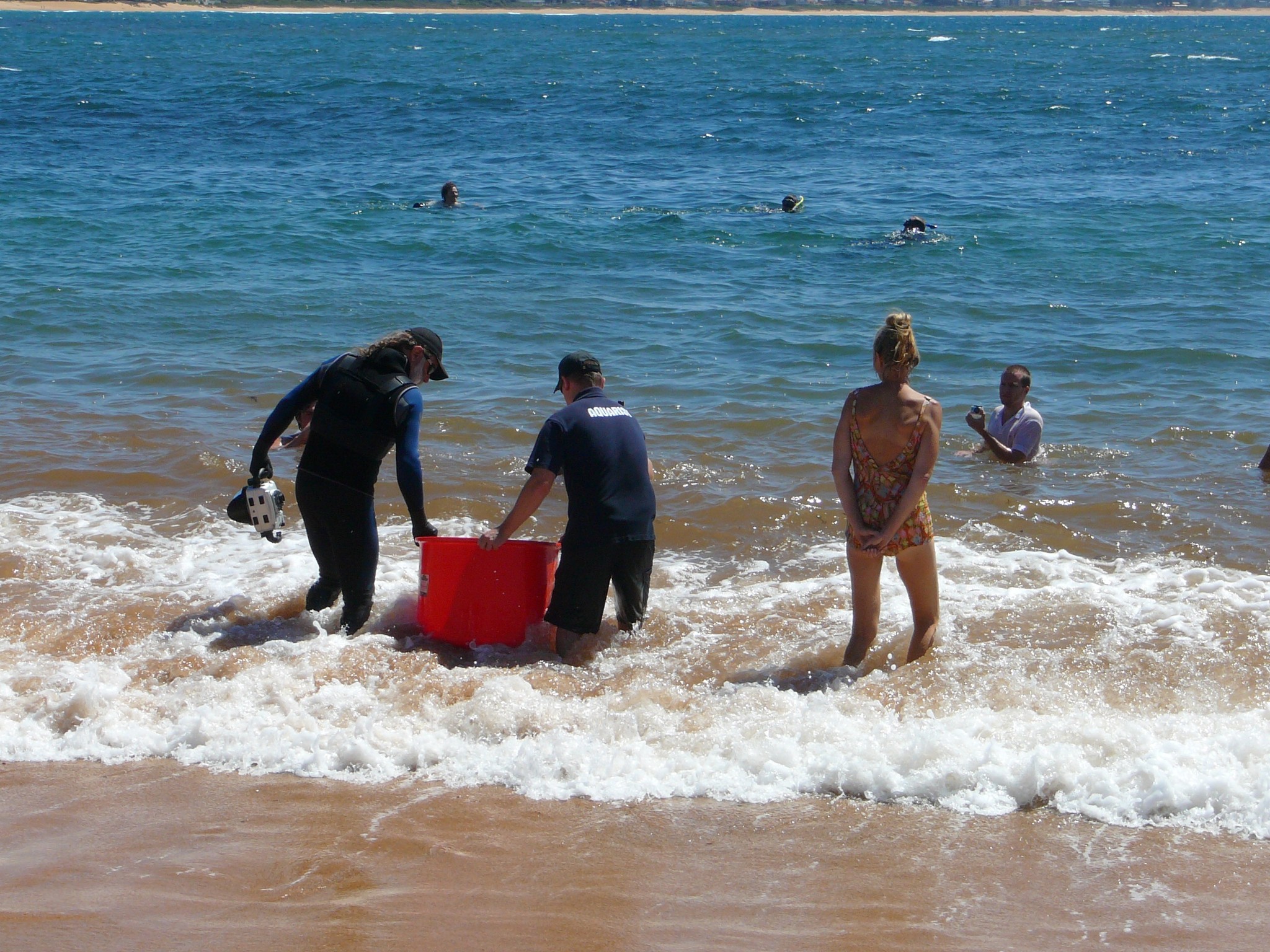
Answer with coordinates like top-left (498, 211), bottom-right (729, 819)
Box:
top-left (478, 347), bottom-right (657, 658)
top-left (267, 399), bottom-right (319, 453)
top-left (428, 184), bottom-right (460, 207)
top-left (903, 217), bottom-right (926, 233)
top-left (830, 311), bottom-right (941, 668)
top-left (250, 327), bottom-right (437, 638)
top-left (782, 194), bottom-right (800, 213)
top-left (954, 363), bottom-right (1043, 464)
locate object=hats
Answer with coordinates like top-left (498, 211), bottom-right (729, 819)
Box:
top-left (407, 326), bottom-right (450, 382)
top-left (552, 348), bottom-right (602, 396)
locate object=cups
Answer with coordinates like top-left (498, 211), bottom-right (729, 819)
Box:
top-left (970, 404), bottom-right (984, 420)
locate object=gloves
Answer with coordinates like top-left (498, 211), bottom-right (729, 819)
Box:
top-left (248, 432), bottom-right (277, 482)
top-left (403, 486), bottom-right (440, 548)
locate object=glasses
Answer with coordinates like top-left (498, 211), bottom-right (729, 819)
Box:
top-left (420, 347), bottom-right (437, 380)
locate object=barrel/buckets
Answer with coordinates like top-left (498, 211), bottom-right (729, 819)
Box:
top-left (409, 526), bottom-right (561, 652)
